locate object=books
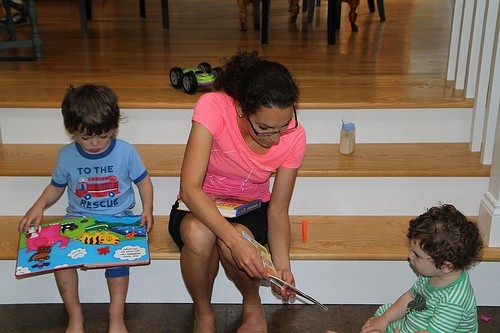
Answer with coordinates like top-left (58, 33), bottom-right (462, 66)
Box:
top-left (174, 191), bottom-right (262, 218)
top-left (242, 230), bottom-right (329, 311)
top-left (13, 215), bottom-right (150, 281)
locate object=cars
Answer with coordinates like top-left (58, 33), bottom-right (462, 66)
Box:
top-left (169, 62), bottom-right (225, 95)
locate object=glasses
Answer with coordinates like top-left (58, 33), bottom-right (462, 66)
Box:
top-left (245, 105), bottom-right (298, 137)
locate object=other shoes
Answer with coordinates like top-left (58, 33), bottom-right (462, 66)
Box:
top-left (0, 13), bottom-right (28, 27)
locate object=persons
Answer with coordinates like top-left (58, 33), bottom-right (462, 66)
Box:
top-left (19, 84), bottom-right (154, 333)
top-left (360, 204), bottom-right (480, 333)
top-left (168, 50), bottom-right (306, 333)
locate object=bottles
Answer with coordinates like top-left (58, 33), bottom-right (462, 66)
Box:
top-left (339, 122), bottom-right (356, 155)
